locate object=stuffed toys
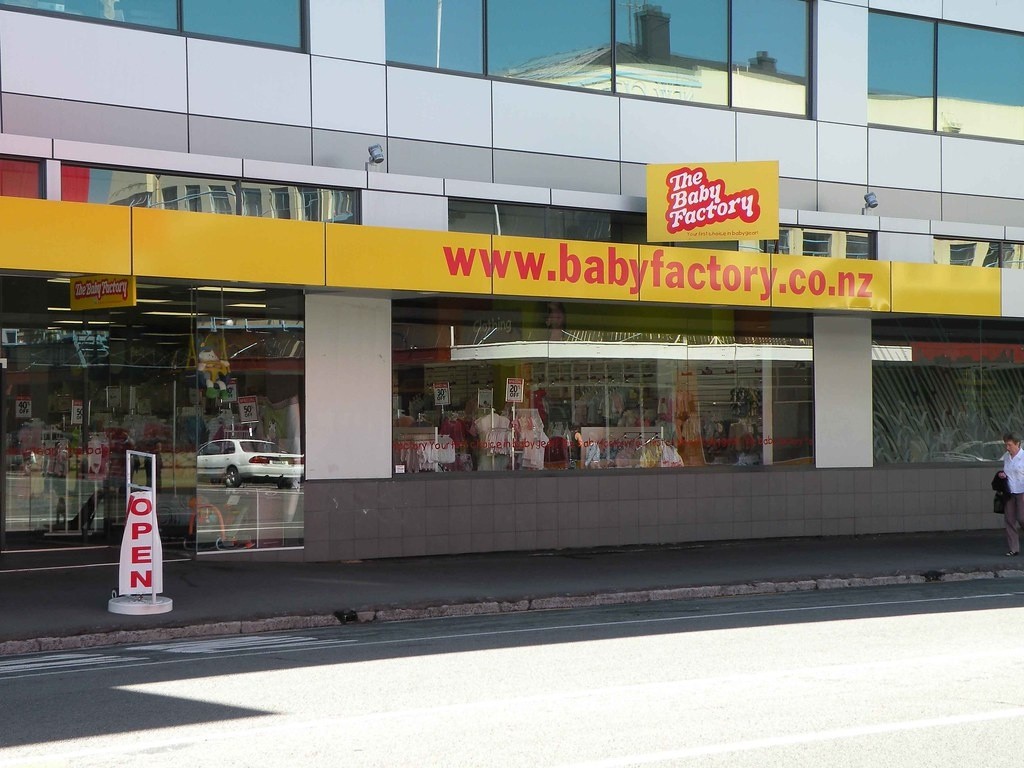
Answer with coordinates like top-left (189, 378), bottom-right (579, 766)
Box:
top-left (198, 348), bottom-right (230, 400)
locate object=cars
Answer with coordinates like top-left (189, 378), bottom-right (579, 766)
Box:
top-left (195, 438), bottom-right (305, 489)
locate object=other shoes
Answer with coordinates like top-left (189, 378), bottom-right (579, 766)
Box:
top-left (1006, 551), bottom-right (1018, 556)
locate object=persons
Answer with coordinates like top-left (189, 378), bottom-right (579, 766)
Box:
top-left (145, 444), bottom-right (163, 492)
top-left (998, 432), bottom-right (1024, 556)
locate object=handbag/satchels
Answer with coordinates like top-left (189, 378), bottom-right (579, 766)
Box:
top-left (994, 493), bottom-right (1005, 514)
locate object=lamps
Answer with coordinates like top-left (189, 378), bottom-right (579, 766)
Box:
top-left (862, 192), bottom-right (878, 215)
top-left (365, 144), bottom-right (384, 172)
